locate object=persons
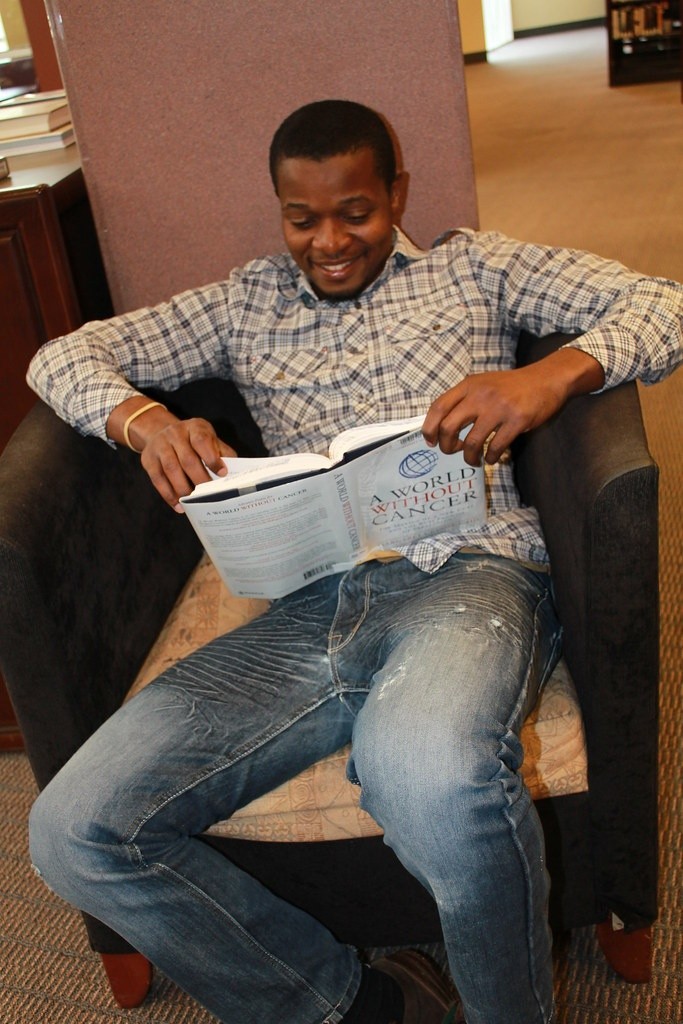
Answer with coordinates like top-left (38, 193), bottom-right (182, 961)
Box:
top-left (22, 98), bottom-right (683, 1024)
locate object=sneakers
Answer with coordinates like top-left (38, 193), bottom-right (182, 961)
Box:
top-left (372, 949), bottom-right (463, 1024)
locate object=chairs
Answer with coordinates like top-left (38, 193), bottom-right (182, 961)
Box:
top-left (1, 309), bottom-right (664, 1013)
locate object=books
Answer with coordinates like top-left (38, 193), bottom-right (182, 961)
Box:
top-left (1, 48), bottom-right (78, 182)
top-left (177, 410), bottom-right (479, 600)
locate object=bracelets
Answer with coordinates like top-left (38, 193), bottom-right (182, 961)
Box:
top-left (124, 401), bottom-right (167, 454)
